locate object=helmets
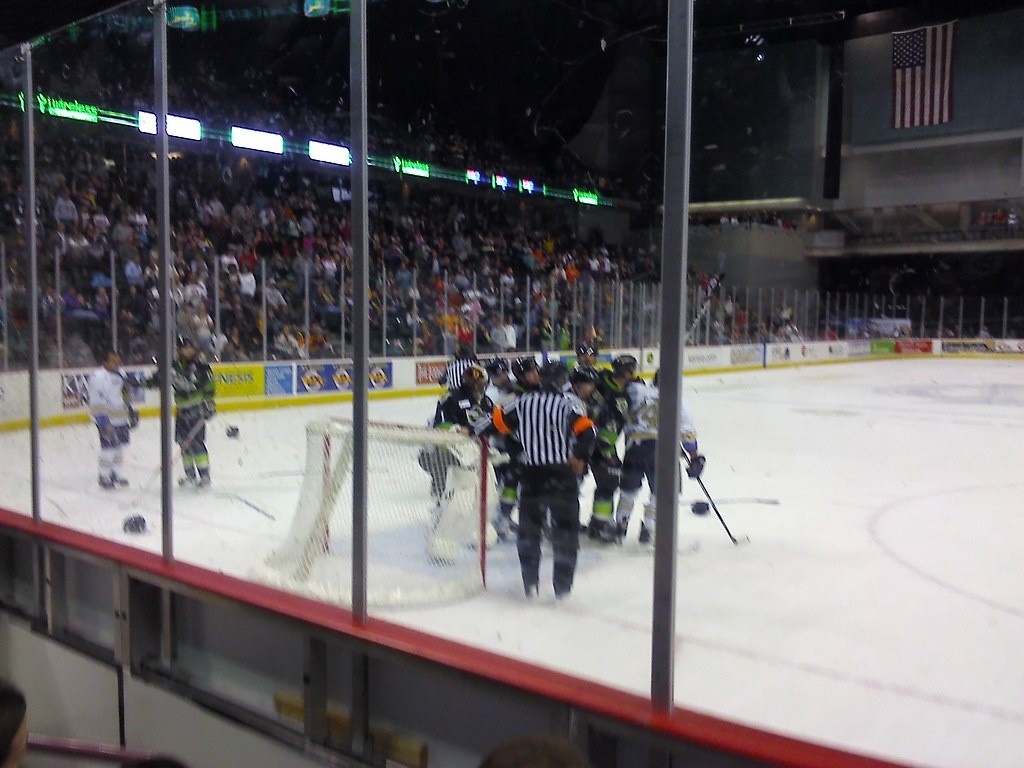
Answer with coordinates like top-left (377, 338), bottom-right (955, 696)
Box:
top-left (176, 334), bottom-right (190, 345)
top-left (452, 340), bottom-right (660, 394)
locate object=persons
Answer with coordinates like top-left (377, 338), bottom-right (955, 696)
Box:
top-left (685, 205), bottom-right (1017, 347)
top-left (0, 26), bottom-right (662, 368)
top-left (416, 342), bottom-right (707, 603)
top-left (88, 348), bottom-right (139, 491)
top-left (126, 336), bottom-right (216, 490)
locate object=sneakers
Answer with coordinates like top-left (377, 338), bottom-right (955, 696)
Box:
top-left (554, 587), bottom-right (572, 610)
top-left (587, 515), bottom-right (615, 543)
top-left (178, 466), bottom-right (196, 485)
top-left (639, 518), bottom-right (655, 543)
top-left (578, 523), bottom-right (587, 533)
top-left (99, 476), bottom-right (115, 491)
top-left (196, 468), bottom-right (211, 490)
top-left (616, 512), bottom-right (628, 536)
top-left (113, 478), bottom-right (128, 487)
top-left (500, 503), bottom-right (519, 533)
top-left (525, 583), bottom-right (539, 603)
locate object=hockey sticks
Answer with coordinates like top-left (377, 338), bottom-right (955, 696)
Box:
top-left (676, 435), bottom-right (753, 546)
top-left (655, 272), bottom-right (726, 365)
top-left (117, 406), bottom-right (213, 512)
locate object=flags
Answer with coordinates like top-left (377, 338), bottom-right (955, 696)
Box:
top-left (891, 20), bottom-right (957, 128)
